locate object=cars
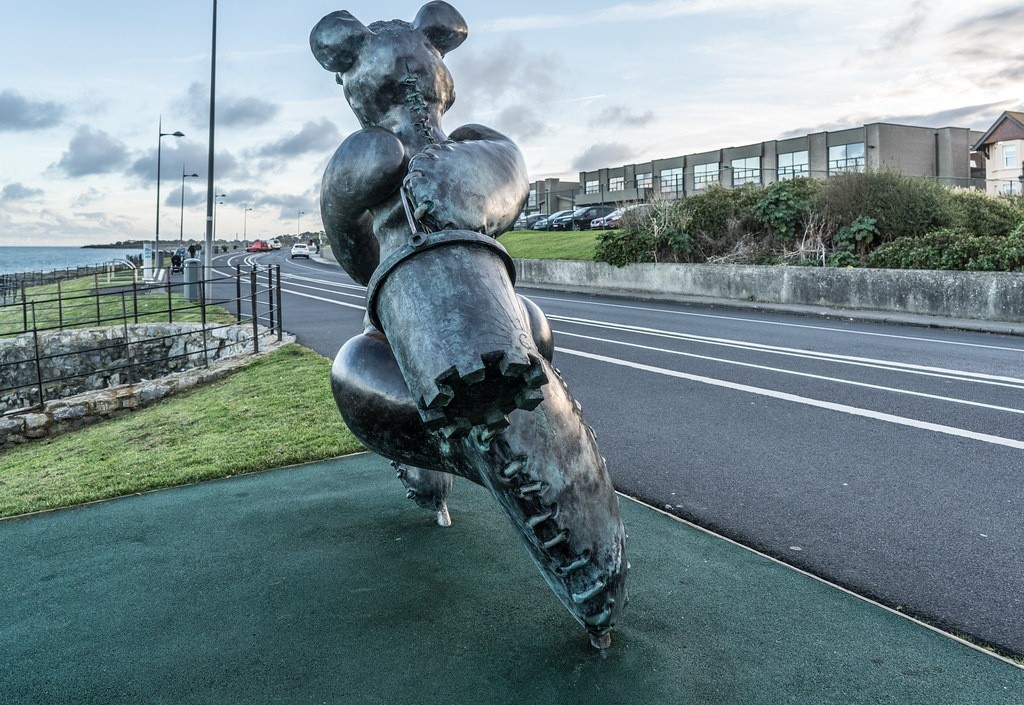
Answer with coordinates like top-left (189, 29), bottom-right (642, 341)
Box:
top-left (513, 214), bottom-right (552, 230)
top-left (291, 243), bottom-right (310, 259)
top-left (590, 204), bottom-right (670, 230)
top-left (532, 210), bottom-right (576, 231)
top-left (245, 239), bottom-right (281, 253)
top-left (553, 206), bottom-right (620, 231)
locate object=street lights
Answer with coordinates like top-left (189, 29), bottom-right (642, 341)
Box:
top-left (298, 212), bottom-right (304, 244)
top-left (245, 208), bottom-right (252, 248)
top-left (180, 174), bottom-right (199, 242)
top-left (214, 194), bottom-right (226, 246)
top-left (156, 132), bottom-right (185, 269)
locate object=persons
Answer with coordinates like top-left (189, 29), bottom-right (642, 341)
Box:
top-left (316, 239), bottom-right (319, 254)
top-left (174, 243), bottom-right (186, 266)
top-left (188, 242), bottom-right (202, 259)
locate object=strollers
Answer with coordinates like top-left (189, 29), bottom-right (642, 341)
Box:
top-left (170, 255), bottom-right (184, 275)
